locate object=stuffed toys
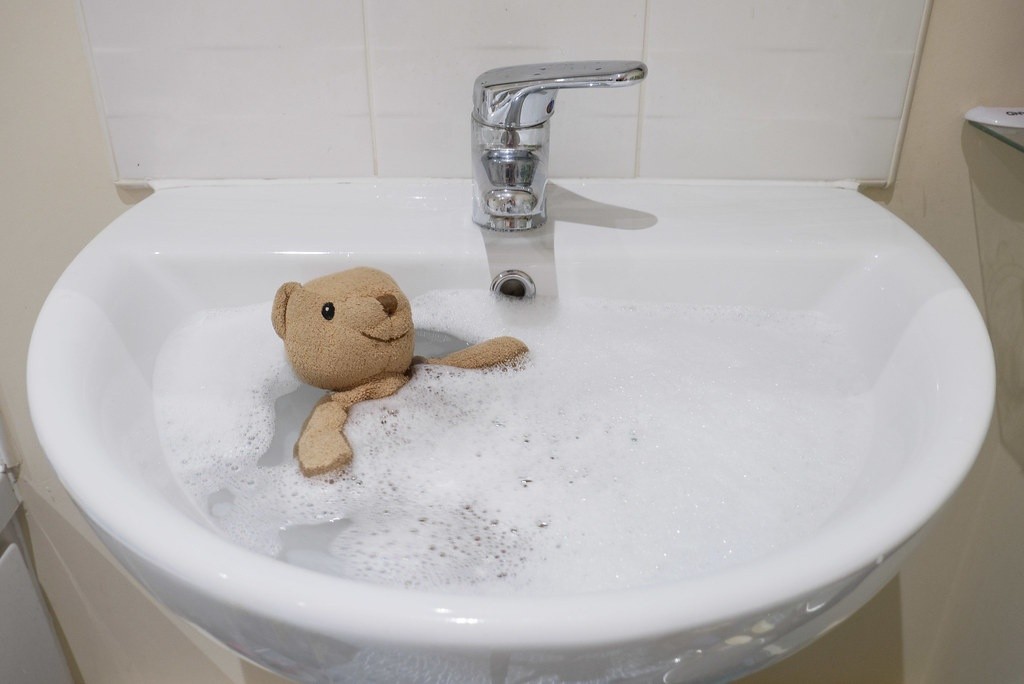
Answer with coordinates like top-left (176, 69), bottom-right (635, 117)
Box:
top-left (271, 265), bottom-right (529, 480)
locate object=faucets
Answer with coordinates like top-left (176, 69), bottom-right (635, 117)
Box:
top-left (462, 60), bottom-right (651, 235)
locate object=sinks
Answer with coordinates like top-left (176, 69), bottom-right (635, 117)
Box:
top-left (23, 176), bottom-right (999, 681)
